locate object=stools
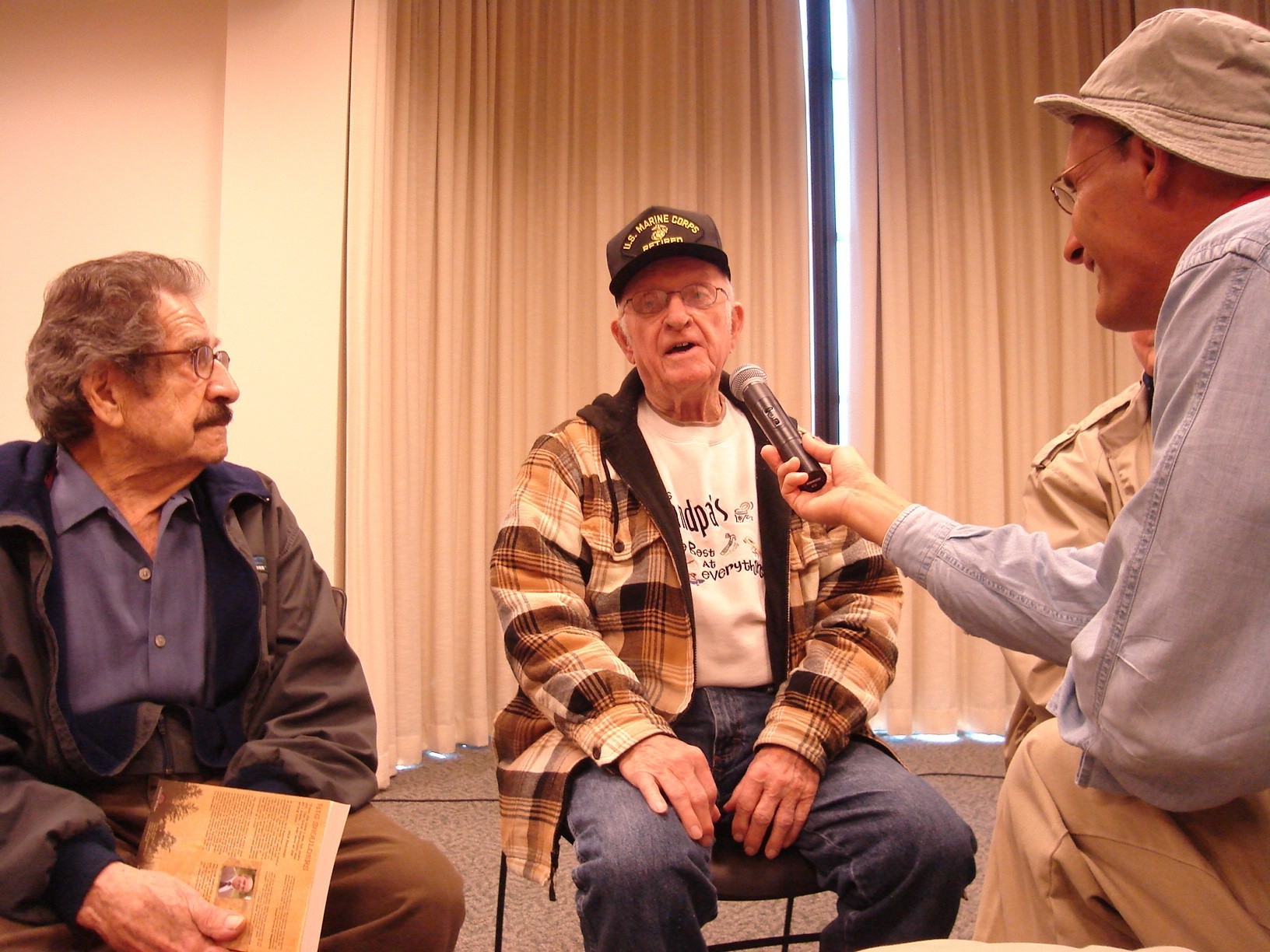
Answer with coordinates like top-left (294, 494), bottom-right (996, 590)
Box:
top-left (493, 810), bottom-right (831, 952)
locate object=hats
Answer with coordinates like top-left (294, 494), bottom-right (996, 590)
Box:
top-left (1032, 7), bottom-right (1270, 183)
top-left (605, 205), bottom-right (732, 305)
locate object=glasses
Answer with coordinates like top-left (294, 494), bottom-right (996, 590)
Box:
top-left (112, 344), bottom-right (233, 380)
top-left (620, 284), bottom-right (730, 319)
top-left (1050, 129), bottom-right (1137, 216)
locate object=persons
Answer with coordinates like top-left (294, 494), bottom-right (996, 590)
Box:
top-left (488, 207), bottom-right (979, 952)
top-left (218, 866), bottom-right (253, 900)
top-left (0, 249), bottom-right (468, 952)
top-left (761, 8), bottom-right (1270, 952)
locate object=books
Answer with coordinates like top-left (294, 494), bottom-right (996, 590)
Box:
top-left (132, 779), bottom-right (350, 952)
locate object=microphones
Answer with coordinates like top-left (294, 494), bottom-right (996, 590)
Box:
top-left (729, 364), bottom-right (827, 493)
